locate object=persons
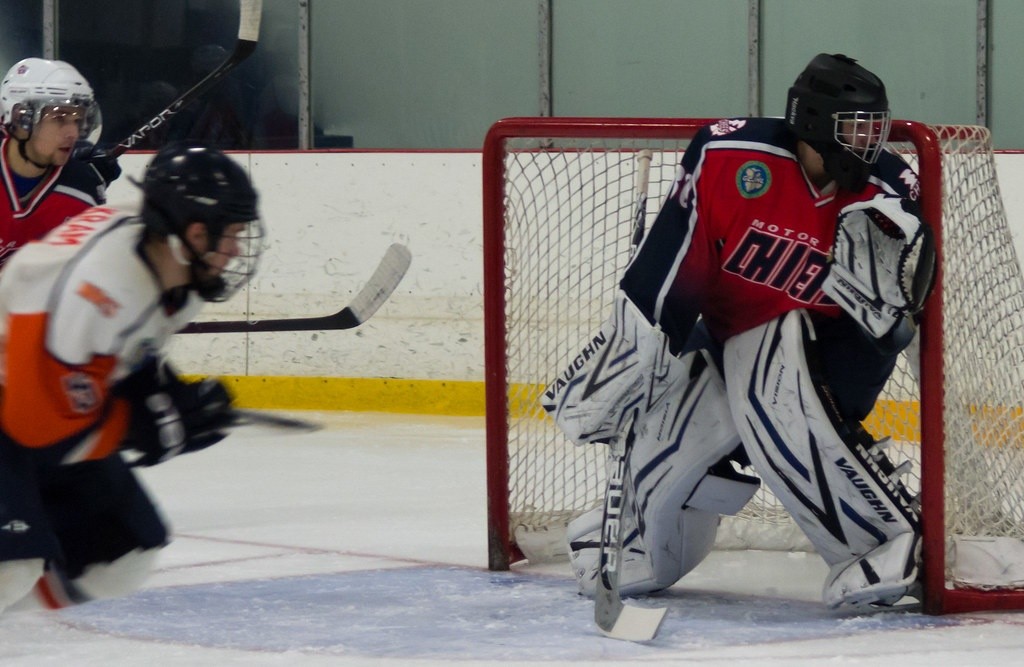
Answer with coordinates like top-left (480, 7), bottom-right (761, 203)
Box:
top-left (0, 57), bottom-right (103, 270)
top-left (1, 148), bottom-right (262, 616)
top-left (562, 56), bottom-right (954, 606)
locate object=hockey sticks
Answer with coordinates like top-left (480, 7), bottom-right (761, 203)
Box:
top-left (595, 150), bottom-right (664, 643)
top-left (179, 239), bottom-right (412, 334)
top-left (87, 40), bottom-right (256, 161)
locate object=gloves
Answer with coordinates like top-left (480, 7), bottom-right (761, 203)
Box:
top-left (132, 374), bottom-right (233, 468)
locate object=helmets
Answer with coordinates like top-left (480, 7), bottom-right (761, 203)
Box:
top-left (785, 51), bottom-right (889, 150)
top-left (3, 56), bottom-right (95, 125)
top-left (140, 140), bottom-right (260, 233)
